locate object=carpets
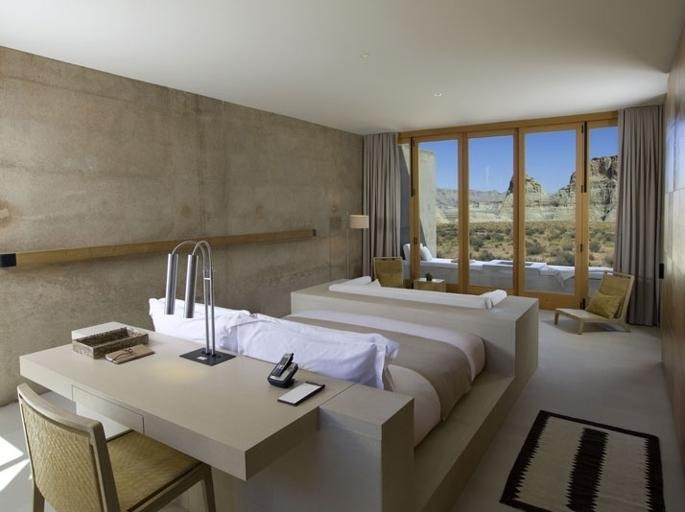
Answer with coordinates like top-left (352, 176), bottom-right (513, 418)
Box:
top-left (499, 407), bottom-right (670, 511)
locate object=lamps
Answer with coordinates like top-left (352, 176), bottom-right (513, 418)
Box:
top-left (348, 214), bottom-right (370, 230)
top-left (163, 241), bottom-right (237, 366)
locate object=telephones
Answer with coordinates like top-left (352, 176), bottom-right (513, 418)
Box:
top-left (268, 352), bottom-right (298, 387)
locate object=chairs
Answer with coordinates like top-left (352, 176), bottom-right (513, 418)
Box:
top-left (553, 269), bottom-right (637, 337)
top-left (15, 380), bottom-right (219, 512)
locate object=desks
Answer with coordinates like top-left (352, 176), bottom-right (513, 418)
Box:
top-left (18, 331), bottom-right (320, 485)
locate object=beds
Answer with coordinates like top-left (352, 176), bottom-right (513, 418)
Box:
top-left (13, 276), bottom-right (541, 512)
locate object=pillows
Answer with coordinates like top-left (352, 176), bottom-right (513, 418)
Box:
top-left (585, 289), bottom-right (625, 321)
top-left (147, 294), bottom-right (401, 392)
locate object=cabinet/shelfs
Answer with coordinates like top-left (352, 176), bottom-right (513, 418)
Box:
top-left (413, 277), bottom-right (447, 293)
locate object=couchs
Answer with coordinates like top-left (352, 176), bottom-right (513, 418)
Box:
top-left (290, 277), bottom-right (539, 378)
